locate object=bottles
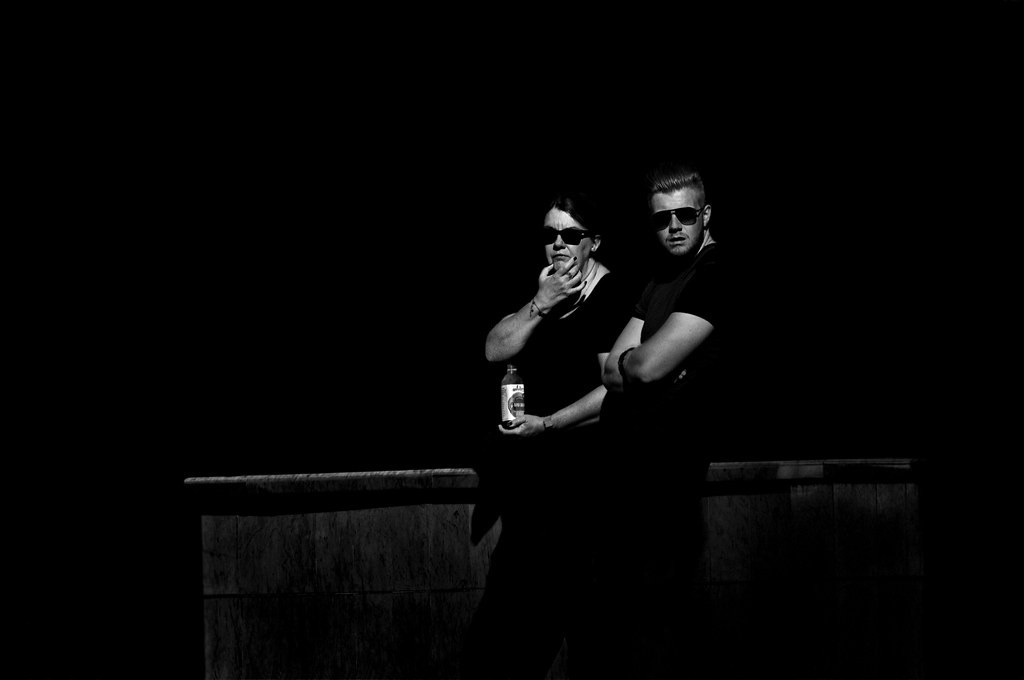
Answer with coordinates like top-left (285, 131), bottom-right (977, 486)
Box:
top-left (500, 365), bottom-right (526, 429)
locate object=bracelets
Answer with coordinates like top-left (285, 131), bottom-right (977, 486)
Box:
top-left (618, 348), bottom-right (634, 375)
top-left (532, 301), bottom-right (546, 319)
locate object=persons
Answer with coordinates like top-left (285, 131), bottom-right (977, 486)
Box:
top-left (474, 191), bottom-right (622, 680)
top-left (566, 161), bottom-right (748, 680)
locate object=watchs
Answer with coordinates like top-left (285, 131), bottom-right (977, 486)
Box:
top-left (542, 416), bottom-right (553, 431)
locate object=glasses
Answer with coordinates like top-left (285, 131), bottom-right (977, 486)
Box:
top-left (650, 207), bottom-right (703, 231)
top-left (541, 226), bottom-right (591, 245)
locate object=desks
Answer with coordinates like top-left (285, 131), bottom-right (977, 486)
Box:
top-left (183, 457), bottom-right (922, 680)
